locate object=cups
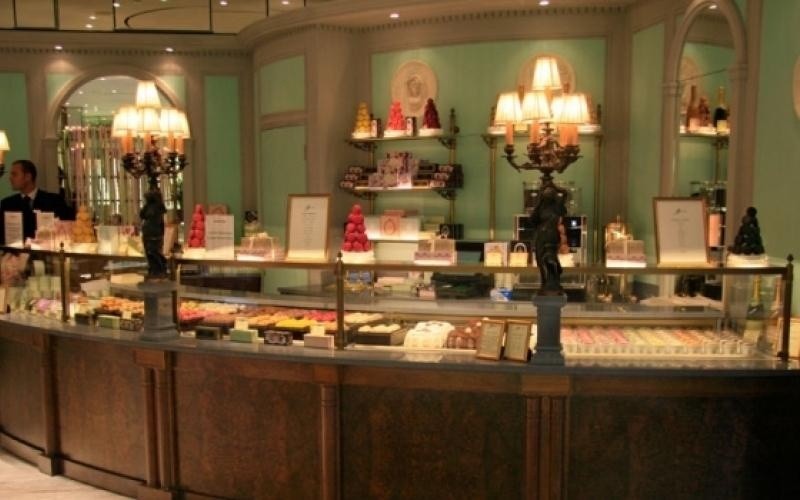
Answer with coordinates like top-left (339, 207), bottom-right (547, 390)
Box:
top-left (24, 260), bottom-right (61, 298)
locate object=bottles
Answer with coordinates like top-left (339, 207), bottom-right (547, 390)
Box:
top-left (688, 178), bottom-right (728, 253)
top-left (744, 272), bottom-right (785, 354)
top-left (684, 78), bottom-right (732, 133)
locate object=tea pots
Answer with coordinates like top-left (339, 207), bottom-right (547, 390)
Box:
top-left (94, 225), bottom-right (131, 256)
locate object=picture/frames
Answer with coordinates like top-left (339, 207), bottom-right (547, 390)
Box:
top-left (652, 196), bottom-right (710, 265)
top-left (285, 193), bottom-right (332, 262)
top-left (503, 320), bottom-right (533, 362)
top-left (474, 320), bottom-right (504, 361)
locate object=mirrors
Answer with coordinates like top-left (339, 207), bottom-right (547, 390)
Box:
top-left (43, 64), bottom-right (196, 225)
top-left (660, 1), bottom-right (748, 262)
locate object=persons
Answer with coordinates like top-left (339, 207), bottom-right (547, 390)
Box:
top-left (0, 159), bottom-right (72, 247)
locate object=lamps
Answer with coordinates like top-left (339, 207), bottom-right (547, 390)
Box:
top-left (491, 56), bottom-right (601, 295)
top-left (111, 79), bottom-right (191, 281)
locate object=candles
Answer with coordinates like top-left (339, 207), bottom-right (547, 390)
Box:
top-left (0, 130), bottom-right (11, 165)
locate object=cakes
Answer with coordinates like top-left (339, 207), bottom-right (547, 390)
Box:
top-left (188, 204), bottom-right (204, 248)
top-left (73, 204), bottom-right (95, 242)
top-left (422, 98), bottom-right (441, 128)
top-left (730, 207), bottom-right (764, 255)
top-left (354, 100), bottom-right (371, 132)
top-left (387, 101), bottom-right (405, 130)
top-left (342, 203), bottom-right (372, 251)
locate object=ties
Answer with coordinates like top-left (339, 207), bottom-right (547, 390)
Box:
top-left (24, 196), bottom-right (30, 205)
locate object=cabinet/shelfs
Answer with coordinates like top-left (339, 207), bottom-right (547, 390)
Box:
top-left (0, 241), bottom-right (800, 500)
top-left (675, 129), bottom-right (729, 247)
top-left (347, 108), bottom-right (456, 238)
top-left (482, 104), bottom-right (602, 264)
